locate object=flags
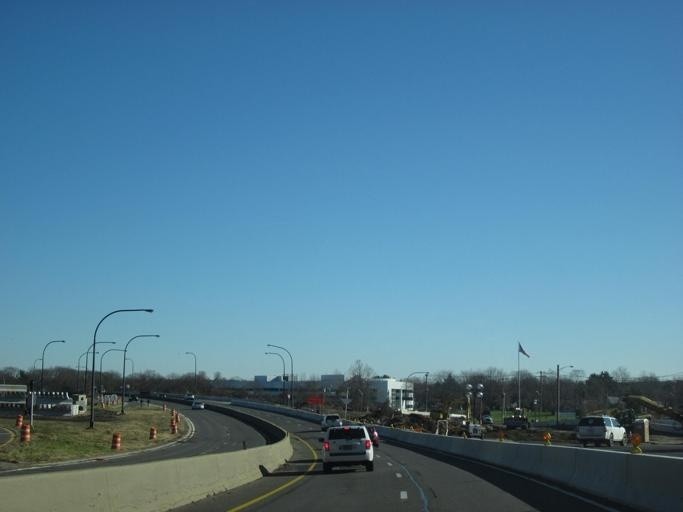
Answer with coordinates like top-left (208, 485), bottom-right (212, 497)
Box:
top-left (519, 343), bottom-right (530, 359)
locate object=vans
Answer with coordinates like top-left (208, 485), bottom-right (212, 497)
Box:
top-left (158, 393), bottom-right (169, 401)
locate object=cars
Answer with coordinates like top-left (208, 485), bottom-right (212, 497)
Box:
top-left (184, 393), bottom-right (204, 410)
top-left (318, 413), bottom-right (379, 473)
top-left (482, 415), bottom-right (493, 424)
top-left (576, 416), bottom-right (627, 447)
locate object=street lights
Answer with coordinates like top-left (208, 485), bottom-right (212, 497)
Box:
top-left (265, 344), bottom-right (293, 408)
top-left (34, 339), bottom-right (66, 396)
top-left (465, 383), bottom-right (473, 420)
top-left (405, 371), bottom-right (430, 408)
top-left (77, 309), bottom-right (159, 429)
top-left (476, 383), bottom-right (484, 424)
top-left (185, 352), bottom-right (197, 384)
top-left (557, 364), bottom-right (574, 423)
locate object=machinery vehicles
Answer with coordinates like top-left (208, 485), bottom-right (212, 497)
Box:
top-left (504, 408), bottom-right (531, 429)
top-left (576, 395), bottom-right (683, 431)
top-left (468, 424), bottom-right (487, 439)
